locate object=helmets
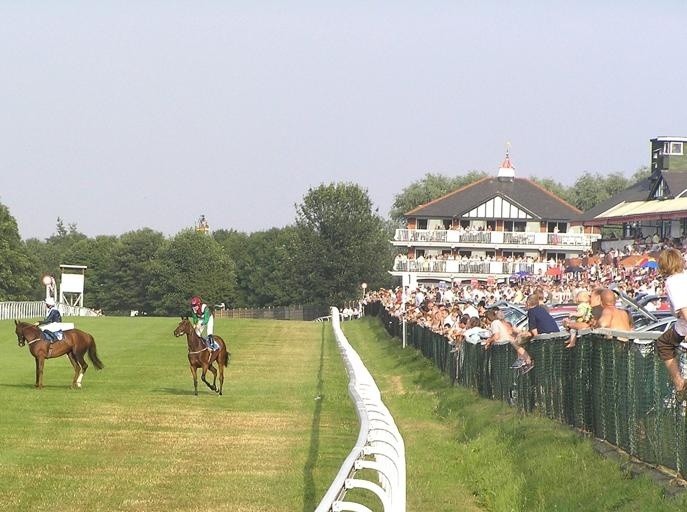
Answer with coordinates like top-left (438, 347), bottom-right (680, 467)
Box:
top-left (190, 296), bottom-right (202, 305)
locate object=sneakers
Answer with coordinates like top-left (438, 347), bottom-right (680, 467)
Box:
top-left (510, 358), bottom-right (526, 370)
top-left (675, 379), bottom-right (687, 406)
top-left (521, 360), bottom-right (535, 374)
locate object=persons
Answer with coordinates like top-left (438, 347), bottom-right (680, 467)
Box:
top-left (34, 298), bottom-right (61, 344)
top-left (190, 297), bottom-right (216, 350)
top-left (345, 226), bottom-right (686, 405)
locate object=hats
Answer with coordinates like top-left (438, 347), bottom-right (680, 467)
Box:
top-left (44, 297), bottom-right (55, 307)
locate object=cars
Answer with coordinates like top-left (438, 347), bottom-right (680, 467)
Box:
top-left (476, 286), bottom-right (678, 395)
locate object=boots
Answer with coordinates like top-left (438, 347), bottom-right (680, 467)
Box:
top-left (207, 335), bottom-right (215, 349)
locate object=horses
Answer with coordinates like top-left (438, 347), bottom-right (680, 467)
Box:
top-left (173, 315), bottom-right (231, 396)
top-left (13, 318), bottom-right (105, 389)
